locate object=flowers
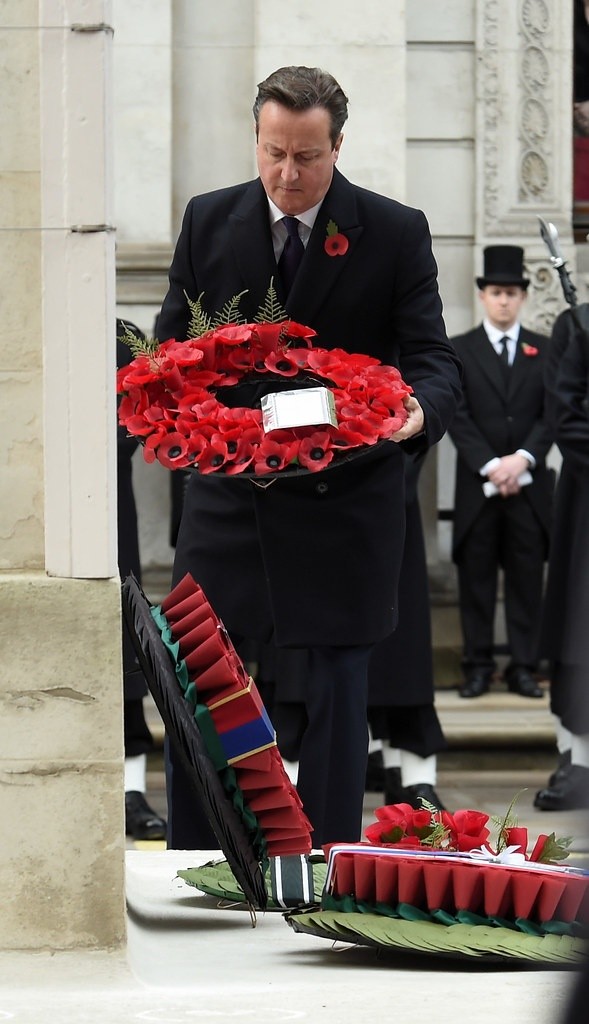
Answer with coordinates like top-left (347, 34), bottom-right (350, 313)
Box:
top-left (521, 342), bottom-right (539, 357)
top-left (325, 221), bottom-right (349, 258)
top-left (361, 786), bottom-right (575, 866)
top-left (117, 272), bottom-right (414, 477)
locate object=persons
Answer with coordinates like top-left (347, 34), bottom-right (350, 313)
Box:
top-left (153, 64), bottom-right (460, 861)
top-left (435, 243), bottom-right (561, 701)
top-left (110, 316), bottom-right (171, 842)
top-left (240, 448), bottom-right (450, 832)
top-left (530, 290), bottom-right (589, 815)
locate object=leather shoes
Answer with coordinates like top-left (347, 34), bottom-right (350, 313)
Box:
top-left (459, 678), bottom-right (490, 698)
top-left (509, 678), bottom-right (545, 698)
top-left (125, 790), bottom-right (168, 840)
top-left (539, 764), bottom-right (589, 811)
top-left (365, 747), bottom-right (447, 810)
top-left (533, 748), bottom-right (571, 807)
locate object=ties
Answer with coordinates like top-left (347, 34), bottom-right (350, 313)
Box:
top-left (499, 336), bottom-right (509, 379)
top-left (276, 216), bottom-right (305, 309)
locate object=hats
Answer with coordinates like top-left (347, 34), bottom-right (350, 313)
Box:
top-left (476, 244), bottom-right (531, 292)
